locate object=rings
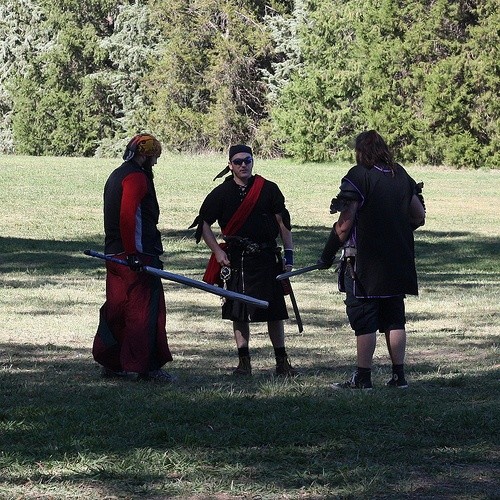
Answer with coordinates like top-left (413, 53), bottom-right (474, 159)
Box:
top-left (135, 261), bottom-right (138, 263)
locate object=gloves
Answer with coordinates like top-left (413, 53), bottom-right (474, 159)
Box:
top-left (317, 222), bottom-right (345, 270)
top-left (128, 255), bottom-right (144, 273)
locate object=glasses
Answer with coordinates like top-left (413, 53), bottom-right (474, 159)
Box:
top-left (231, 157), bottom-right (253, 165)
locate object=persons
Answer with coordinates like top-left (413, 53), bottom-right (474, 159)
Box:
top-left (188, 145), bottom-right (299, 379)
top-left (93, 134), bottom-right (177, 384)
top-left (317, 130), bottom-right (426, 390)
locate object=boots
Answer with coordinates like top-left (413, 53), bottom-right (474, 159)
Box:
top-left (275, 354), bottom-right (299, 375)
top-left (233, 356), bottom-right (251, 375)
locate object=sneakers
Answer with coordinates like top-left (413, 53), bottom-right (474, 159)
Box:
top-left (385, 372), bottom-right (408, 388)
top-left (331, 374), bottom-right (372, 391)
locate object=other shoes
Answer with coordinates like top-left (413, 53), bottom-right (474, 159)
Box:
top-left (102, 366), bottom-right (126, 377)
top-left (138, 368), bottom-right (176, 383)
